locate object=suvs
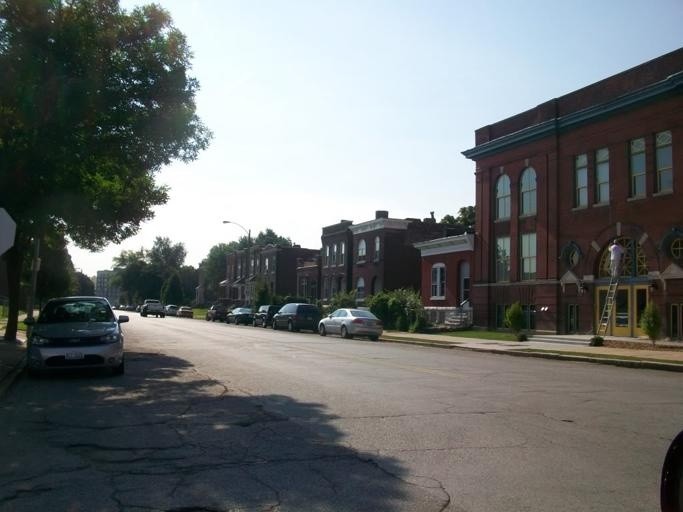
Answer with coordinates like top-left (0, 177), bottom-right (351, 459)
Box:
top-left (138, 298), bottom-right (166, 318)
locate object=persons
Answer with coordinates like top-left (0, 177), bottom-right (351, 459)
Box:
top-left (607, 239), bottom-right (626, 284)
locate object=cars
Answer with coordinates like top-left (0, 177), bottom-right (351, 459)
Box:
top-left (204, 303), bottom-right (384, 341)
top-left (24, 293), bottom-right (130, 375)
top-left (111, 303), bottom-right (194, 319)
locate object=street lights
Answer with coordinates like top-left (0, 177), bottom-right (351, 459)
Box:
top-left (222, 217), bottom-right (252, 302)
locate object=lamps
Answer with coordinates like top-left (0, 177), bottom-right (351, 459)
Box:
top-left (649, 280), bottom-right (658, 293)
top-left (577, 280), bottom-right (588, 296)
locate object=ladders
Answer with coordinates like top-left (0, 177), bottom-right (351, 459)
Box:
top-left (598, 277), bottom-right (620, 336)
